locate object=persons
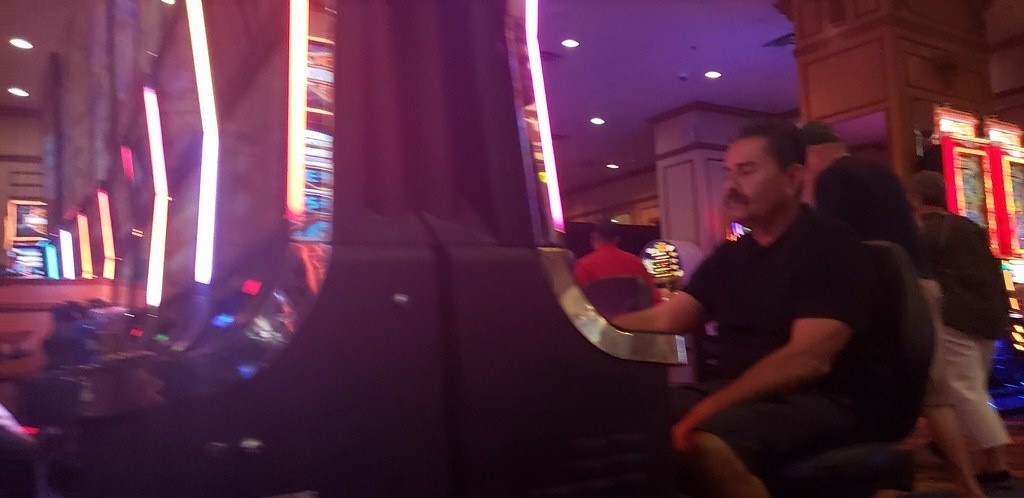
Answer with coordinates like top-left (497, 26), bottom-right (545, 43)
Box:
top-left (571, 220), bottom-right (662, 305)
top-left (900, 171), bottom-right (1023, 498)
top-left (607, 118), bottom-right (906, 498)
top-left (797, 120), bottom-right (986, 497)
